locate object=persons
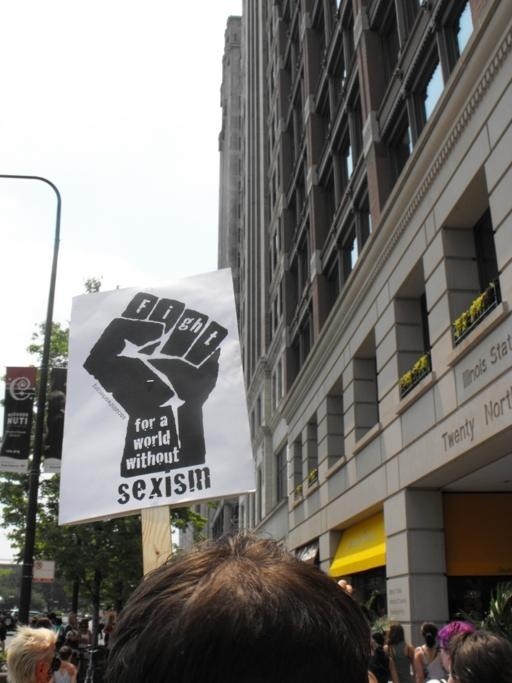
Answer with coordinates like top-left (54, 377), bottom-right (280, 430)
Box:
top-left (368, 621), bottom-right (512, 683)
top-left (0, 611), bottom-right (116, 683)
top-left (104, 532), bottom-right (372, 683)
